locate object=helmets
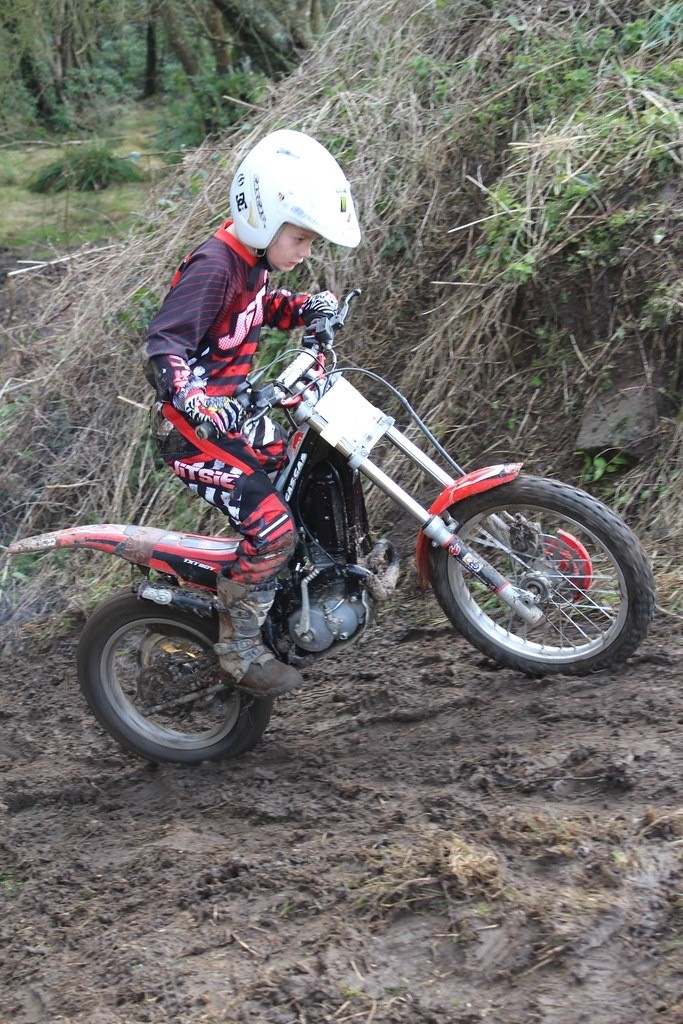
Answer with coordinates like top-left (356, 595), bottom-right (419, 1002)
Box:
top-left (231, 129), bottom-right (361, 250)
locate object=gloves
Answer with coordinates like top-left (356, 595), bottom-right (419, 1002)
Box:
top-left (299, 290), bottom-right (339, 328)
top-left (183, 389), bottom-right (248, 441)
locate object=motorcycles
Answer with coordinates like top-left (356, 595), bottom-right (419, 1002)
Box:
top-left (8, 324), bottom-right (653, 768)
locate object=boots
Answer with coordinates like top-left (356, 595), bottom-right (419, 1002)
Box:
top-left (212, 565), bottom-right (302, 694)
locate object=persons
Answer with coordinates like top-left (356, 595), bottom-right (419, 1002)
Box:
top-left (137, 131), bottom-right (360, 703)
